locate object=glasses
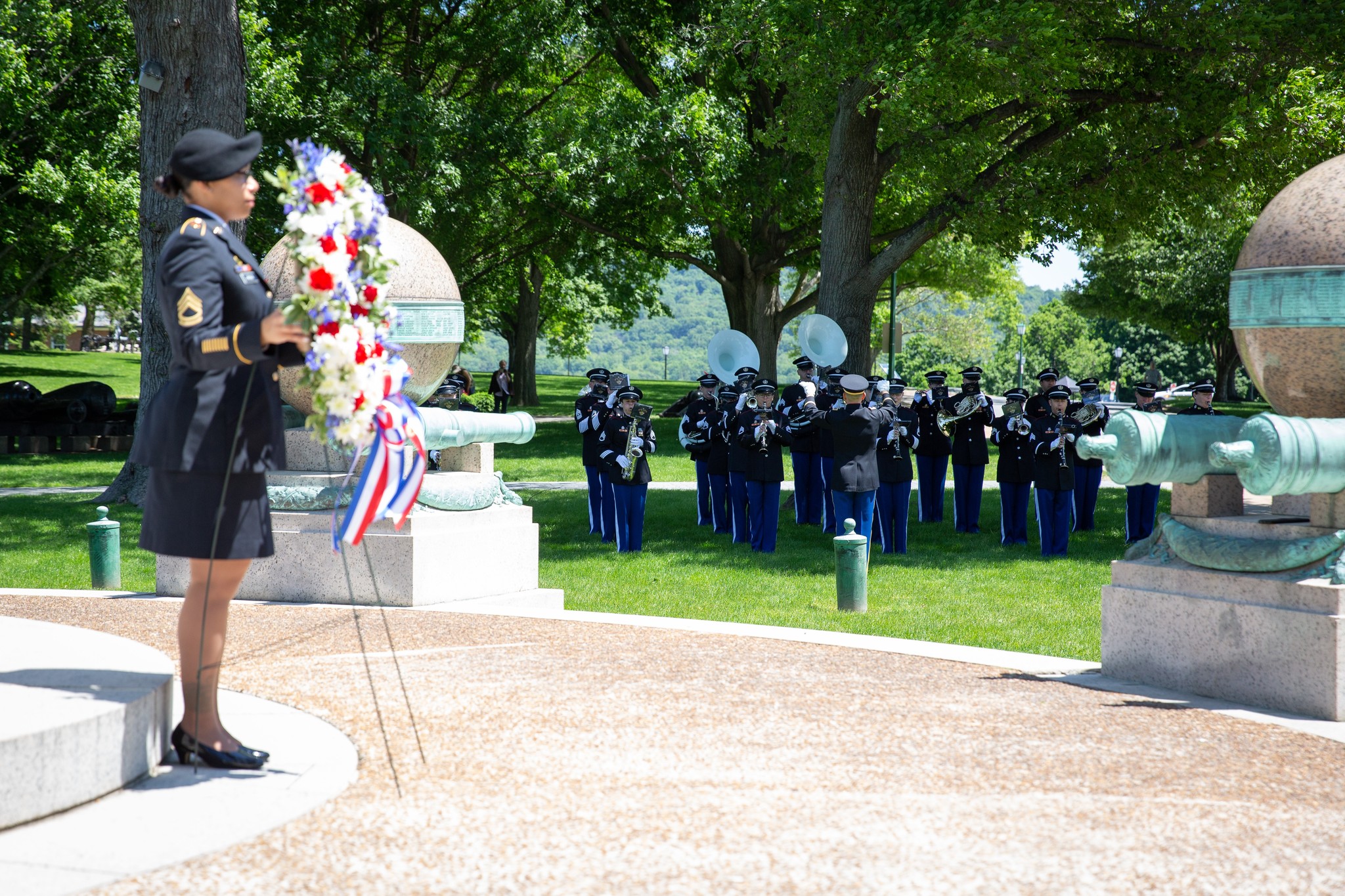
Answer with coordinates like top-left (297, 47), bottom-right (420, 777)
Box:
top-left (798, 368), bottom-right (814, 372)
top-left (890, 393), bottom-right (902, 396)
top-left (238, 171), bottom-right (251, 183)
top-left (831, 381), bottom-right (839, 384)
top-left (865, 388), bottom-right (871, 393)
top-left (930, 383), bottom-right (944, 387)
top-left (757, 393), bottom-right (773, 398)
top-left (590, 381), bottom-right (607, 385)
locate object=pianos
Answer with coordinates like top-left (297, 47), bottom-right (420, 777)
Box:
top-left (915, 390), bottom-right (925, 394)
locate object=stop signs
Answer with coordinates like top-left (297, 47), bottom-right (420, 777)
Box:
top-left (1109, 380), bottom-right (1117, 393)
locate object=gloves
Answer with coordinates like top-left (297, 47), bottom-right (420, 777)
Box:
top-left (697, 417), bottom-right (706, 428)
top-left (1094, 402), bottom-right (1105, 418)
top-left (811, 376), bottom-right (827, 390)
top-left (868, 401), bottom-right (880, 409)
top-left (606, 390), bottom-right (618, 408)
top-left (735, 392), bottom-right (751, 411)
top-left (877, 380), bottom-right (890, 394)
top-left (977, 391), bottom-right (988, 407)
top-left (1017, 418), bottom-right (1031, 429)
top-left (616, 437), bottom-right (644, 468)
top-left (799, 381), bottom-right (816, 397)
top-left (832, 399), bottom-right (845, 409)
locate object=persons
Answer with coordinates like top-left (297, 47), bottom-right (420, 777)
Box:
top-left (1144, 361), bottom-right (1163, 401)
top-left (778, 356), bottom-right (921, 555)
top-left (1120, 383), bottom-right (1165, 547)
top-left (797, 374), bottom-right (897, 578)
top-left (939, 365), bottom-right (996, 535)
top-left (1024, 366), bottom-right (1071, 524)
top-left (991, 387), bottom-right (1038, 547)
top-left (414, 365), bottom-right (476, 470)
top-left (487, 360), bottom-right (514, 414)
top-left (127, 126), bottom-right (313, 772)
top-left (681, 366), bottom-right (794, 554)
top-left (1177, 379), bottom-right (1227, 415)
top-left (1069, 375), bottom-right (1111, 532)
top-left (574, 367), bottom-right (657, 555)
top-left (1029, 383), bottom-right (1085, 559)
top-left (909, 369), bottom-right (952, 525)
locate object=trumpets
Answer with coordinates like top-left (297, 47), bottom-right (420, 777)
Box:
top-left (1015, 414), bottom-right (1030, 436)
top-left (746, 391), bottom-right (758, 409)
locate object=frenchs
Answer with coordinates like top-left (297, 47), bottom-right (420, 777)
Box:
top-left (936, 395), bottom-right (982, 437)
top-left (1070, 403), bottom-right (1101, 427)
top-left (775, 314), bottom-right (849, 431)
top-left (678, 329), bottom-right (761, 454)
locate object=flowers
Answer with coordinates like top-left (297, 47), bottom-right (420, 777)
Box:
top-left (263, 138), bottom-right (411, 448)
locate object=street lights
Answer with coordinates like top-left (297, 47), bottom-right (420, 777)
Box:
top-left (1015, 320), bottom-right (1026, 388)
top-left (1114, 346), bottom-right (1123, 402)
top-left (661, 345), bottom-right (669, 380)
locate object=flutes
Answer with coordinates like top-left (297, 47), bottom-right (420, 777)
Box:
top-left (1057, 409), bottom-right (1069, 470)
top-left (893, 401), bottom-right (903, 462)
top-left (759, 402), bottom-right (768, 456)
top-left (1207, 401), bottom-right (1215, 416)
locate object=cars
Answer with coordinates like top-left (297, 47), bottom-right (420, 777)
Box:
top-left (1100, 394), bottom-right (1120, 402)
top-left (1153, 384), bottom-right (1215, 404)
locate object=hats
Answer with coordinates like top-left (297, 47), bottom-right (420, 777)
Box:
top-left (586, 368), bottom-right (643, 402)
top-left (1191, 378), bottom-right (1216, 393)
top-left (1003, 368), bottom-right (1100, 403)
top-left (696, 366), bottom-right (778, 400)
top-left (432, 373), bottom-right (468, 394)
top-left (1134, 382), bottom-right (1159, 398)
top-left (168, 128), bottom-right (263, 181)
top-left (958, 366), bottom-right (983, 380)
top-left (924, 370), bottom-right (948, 384)
top-left (792, 356), bottom-right (908, 395)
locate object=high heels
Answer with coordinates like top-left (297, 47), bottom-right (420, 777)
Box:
top-left (171, 722), bottom-right (270, 770)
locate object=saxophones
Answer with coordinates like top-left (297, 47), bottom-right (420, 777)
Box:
top-left (621, 407), bottom-right (643, 481)
